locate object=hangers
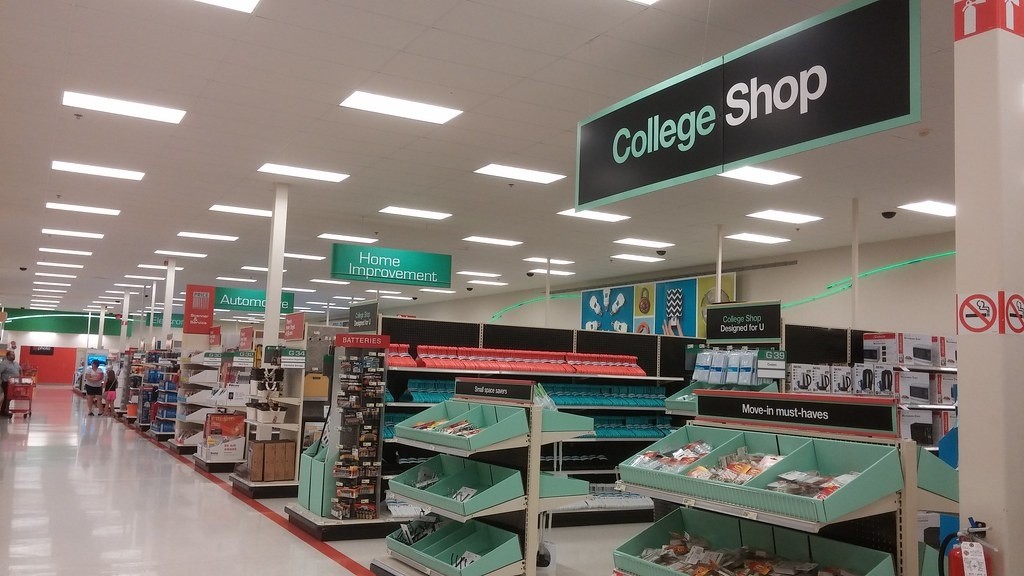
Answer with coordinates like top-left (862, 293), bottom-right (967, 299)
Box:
top-left (384, 413), bottom-right (418, 439)
top-left (383, 491), bottom-right (439, 515)
top-left (547, 483), bottom-right (654, 513)
top-left (385, 343), bottom-right (646, 376)
top-left (385, 379), bottom-right (667, 407)
top-left (581, 416), bottom-right (672, 438)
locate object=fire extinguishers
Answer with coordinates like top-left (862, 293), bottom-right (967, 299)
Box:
top-left (935, 517), bottom-right (999, 576)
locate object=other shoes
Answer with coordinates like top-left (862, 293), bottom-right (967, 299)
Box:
top-left (1, 410), bottom-right (12, 416)
top-left (88, 412), bottom-right (93, 415)
top-left (98, 411), bottom-right (103, 415)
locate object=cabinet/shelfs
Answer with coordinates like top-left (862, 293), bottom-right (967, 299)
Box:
top-left (611, 388), bottom-right (958, 576)
top-left (380, 366), bottom-right (684, 523)
top-left (893, 366), bottom-right (958, 451)
top-left (108, 350), bottom-right (328, 499)
top-left (369, 432), bottom-right (530, 576)
top-left (916, 445), bottom-right (959, 576)
top-left (613, 420), bottom-right (901, 576)
top-left (665, 317), bottom-right (786, 417)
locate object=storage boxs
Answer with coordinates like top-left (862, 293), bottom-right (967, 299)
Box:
top-left (540, 475), bottom-right (589, 497)
top-left (538, 431), bottom-right (595, 514)
top-left (917, 446), bottom-right (959, 503)
top-left (542, 408), bottom-right (594, 432)
top-left (918, 540), bottom-right (948, 576)
top-left (394, 400), bottom-right (530, 451)
top-left (785, 332), bottom-right (958, 447)
top-left (612, 506), bottom-right (896, 576)
top-left (388, 454), bottom-right (525, 516)
top-left (386, 512), bottom-right (523, 576)
top-left (619, 426), bottom-right (905, 523)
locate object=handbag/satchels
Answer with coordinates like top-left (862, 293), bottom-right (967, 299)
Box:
top-left (102, 393), bottom-right (108, 399)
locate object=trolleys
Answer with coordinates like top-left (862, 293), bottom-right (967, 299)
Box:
top-left (8, 376), bottom-right (34, 419)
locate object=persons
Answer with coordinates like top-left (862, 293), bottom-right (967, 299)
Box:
top-left (85, 360), bottom-right (104, 416)
top-left (101, 370), bottom-right (118, 417)
top-left (0, 351), bottom-right (21, 416)
